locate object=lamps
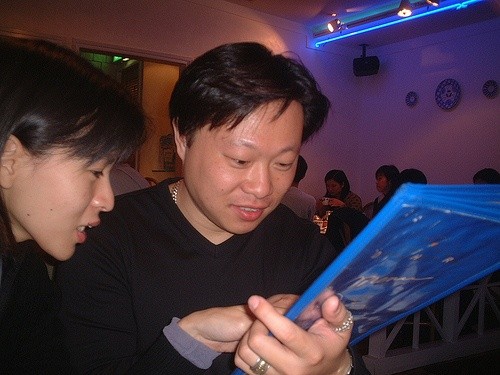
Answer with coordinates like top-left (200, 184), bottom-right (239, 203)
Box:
top-left (426, 0), bottom-right (440, 8)
top-left (396, 0), bottom-right (413, 18)
top-left (327, 18), bottom-right (349, 33)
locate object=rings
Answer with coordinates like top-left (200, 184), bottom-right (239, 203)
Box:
top-left (250, 359), bottom-right (270, 375)
top-left (336, 311), bottom-right (354, 332)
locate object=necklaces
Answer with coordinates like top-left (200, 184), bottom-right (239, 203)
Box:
top-left (172, 178), bottom-right (182, 204)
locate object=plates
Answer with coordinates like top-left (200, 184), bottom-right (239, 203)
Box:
top-left (482, 79), bottom-right (498, 97)
top-left (405, 91), bottom-right (417, 106)
top-left (435, 79), bottom-right (462, 111)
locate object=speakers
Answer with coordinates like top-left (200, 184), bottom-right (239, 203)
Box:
top-left (353, 56), bottom-right (380, 76)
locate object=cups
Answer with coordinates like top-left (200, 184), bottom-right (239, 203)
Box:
top-left (313, 217), bottom-right (328, 230)
top-left (321, 197), bottom-right (330, 205)
top-left (326, 211), bottom-right (332, 220)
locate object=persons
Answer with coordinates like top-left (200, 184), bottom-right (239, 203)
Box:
top-left (473, 168), bottom-right (500, 183)
top-left (363, 165), bottom-right (399, 219)
top-left (315, 170), bottom-right (361, 219)
top-left (61, 42), bottom-right (371, 375)
top-left (109, 163), bottom-right (150, 197)
top-left (377, 169), bottom-right (427, 212)
top-left (0, 38), bottom-right (148, 374)
top-left (279, 155), bottom-right (317, 222)
